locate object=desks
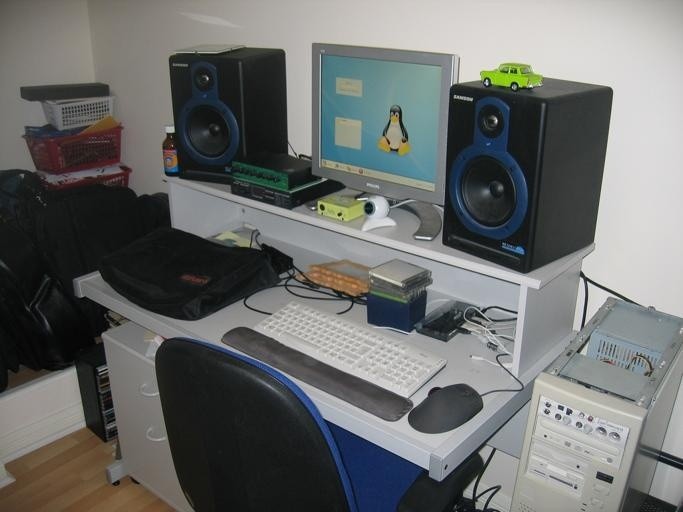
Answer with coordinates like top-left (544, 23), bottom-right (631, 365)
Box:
top-left (70, 154), bottom-right (596, 511)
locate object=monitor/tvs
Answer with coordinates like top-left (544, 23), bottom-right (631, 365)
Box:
top-left (306, 43), bottom-right (459, 241)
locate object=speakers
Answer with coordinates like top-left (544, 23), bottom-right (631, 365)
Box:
top-left (169, 47), bottom-right (287, 185)
top-left (442, 77), bottom-right (613, 274)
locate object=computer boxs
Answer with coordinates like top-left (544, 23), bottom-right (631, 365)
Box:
top-left (509, 296), bottom-right (683, 512)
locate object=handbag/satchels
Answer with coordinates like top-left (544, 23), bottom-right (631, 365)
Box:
top-left (100, 224), bottom-right (278, 320)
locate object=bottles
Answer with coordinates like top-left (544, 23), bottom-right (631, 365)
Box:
top-left (161, 125), bottom-right (180, 177)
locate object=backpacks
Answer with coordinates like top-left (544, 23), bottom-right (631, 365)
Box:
top-left (0, 167), bottom-right (141, 395)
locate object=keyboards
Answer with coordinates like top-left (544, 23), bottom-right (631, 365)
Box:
top-left (254, 301), bottom-right (447, 398)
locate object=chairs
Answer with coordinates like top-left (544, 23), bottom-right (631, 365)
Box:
top-left (151, 336), bottom-right (483, 511)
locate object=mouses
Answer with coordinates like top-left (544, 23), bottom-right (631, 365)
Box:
top-left (408, 384), bottom-right (483, 433)
